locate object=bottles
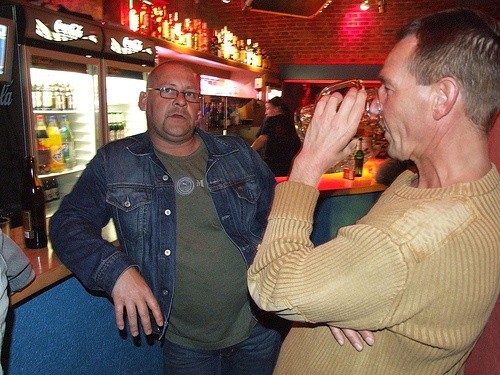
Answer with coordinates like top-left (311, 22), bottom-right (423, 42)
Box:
top-left (31, 82), bottom-right (75, 111)
top-left (21, 157), bottom-right (48, 249)
top-left (205, 101), bottom-right (240, 131)
top-left (107, 111), bottom-right (125, 143)
top-left (58, 114), bottom-right (75, 169)
top-left (127, 0), bottom-right (272, 70)
top-left (51, 178), bottom-right (60, 200)
top-left (35, 114), bottom-right (51, 173)
top-left (43, 178), bottom-right (53, 202)
top-left (354, 138), bottom-right (364, 176)
top-left (46, 116), bottom-right (64, 172)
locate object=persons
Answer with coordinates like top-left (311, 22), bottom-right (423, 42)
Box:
top-left (243, 6), bottom-right (500, 374)
top-left (47, 59), bottom-right (315, 375)
top-left (246, 95), bottom-right (302, 179)
top-left (0, 228), bottom-right (36, 375)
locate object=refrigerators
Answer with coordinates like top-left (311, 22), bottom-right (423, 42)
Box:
top-left (103, 21), bottom-right (156, 147)
top-left (0, 0), bottom-right (103, 218)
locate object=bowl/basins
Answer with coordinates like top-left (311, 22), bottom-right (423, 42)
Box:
top-left (241, 119), bottom-right (253, 125)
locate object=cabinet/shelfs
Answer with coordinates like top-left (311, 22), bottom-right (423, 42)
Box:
top-left (200, 91), bottom-right (265, 146)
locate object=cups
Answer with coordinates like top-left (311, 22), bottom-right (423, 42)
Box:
top-left (0, 216), bottom-right (10, 237)
top-left (294, 77), bottom-right (387, 145)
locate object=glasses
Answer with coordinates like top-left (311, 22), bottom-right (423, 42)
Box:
top-left (146, 87), bottom-right (203, 103)
